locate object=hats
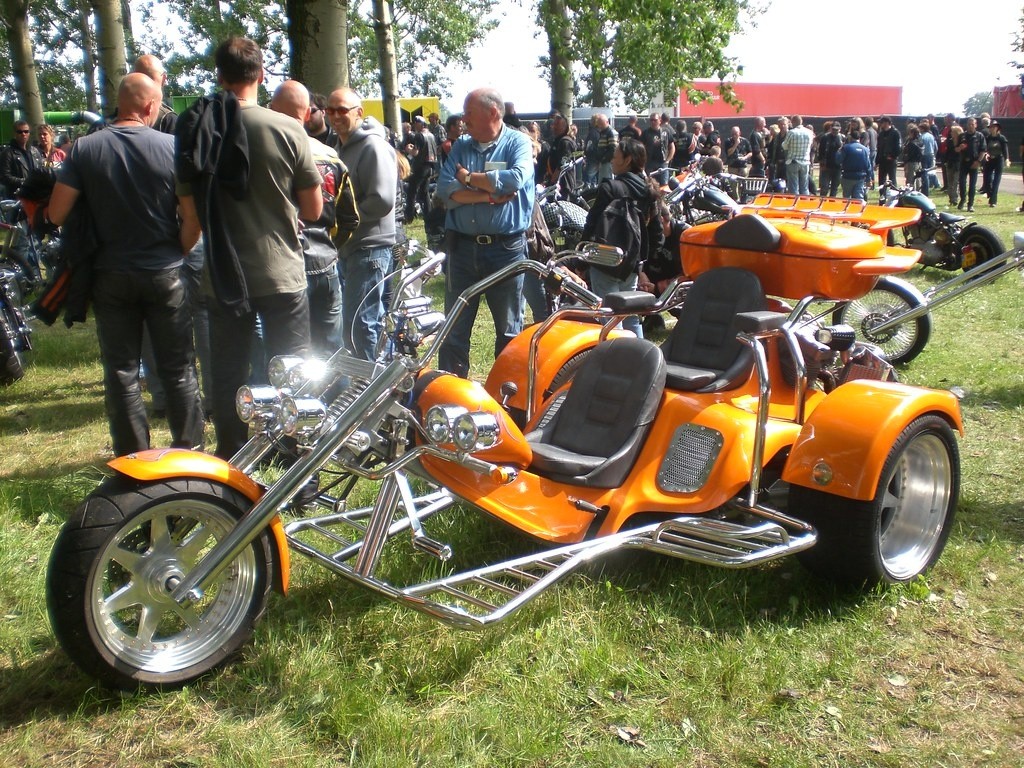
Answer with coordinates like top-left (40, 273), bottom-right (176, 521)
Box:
top-left (414, 115), bottom-right (427, 126)
top-left (877, 115), bottom-right (892, 122)
top-left (850, 130), bottom-right (860, 140)
top-left (985, 119), bottom-right (1000, 127)
top-left (830, 121), bottom-right (842, 130)
top-left (946, 113), bottom-right (957, 121)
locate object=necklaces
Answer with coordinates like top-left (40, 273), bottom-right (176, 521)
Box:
top-left (237, 98), bottom-right (253, 102)
top-left (112, 117), bottom-right (144, 125)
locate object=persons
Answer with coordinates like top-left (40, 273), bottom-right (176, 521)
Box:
top-left (0, 37), bottom-right (1024, 514)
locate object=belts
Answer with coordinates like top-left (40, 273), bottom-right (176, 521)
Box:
top-left (455, 232), bottom-right (521, 244)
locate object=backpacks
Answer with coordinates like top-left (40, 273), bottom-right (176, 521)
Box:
top-left (594, 178), bottom-right (643, 283)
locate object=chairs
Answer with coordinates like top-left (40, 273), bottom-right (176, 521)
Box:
top-left (524, 336), bottom-right (669, 490)
top-left (659, 265), bottom-right (764, 392)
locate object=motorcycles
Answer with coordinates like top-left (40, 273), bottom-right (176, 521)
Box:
top-left (876, 179), bottom-right (1005, 285)
top-left (46, 156), bottom-right (1024, 695)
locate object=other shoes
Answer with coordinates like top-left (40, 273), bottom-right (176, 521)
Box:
top-left (967, 205), bottom-right (975, 212)
top-left (990, 204), bottom-right (996, 207)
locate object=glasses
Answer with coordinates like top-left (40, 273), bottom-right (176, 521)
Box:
top-left (311, 108), bottom-right (320, 114)
top-left (15, 130), bottom-right (31, 133)
top-left (325, 106), bottom-right (359, 114)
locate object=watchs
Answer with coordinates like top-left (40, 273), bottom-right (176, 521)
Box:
top-left (465, 172), bottom-right (472, 187)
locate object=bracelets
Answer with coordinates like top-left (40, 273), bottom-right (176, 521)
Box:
top-left (489, 192), bottom-right (494, 205)
